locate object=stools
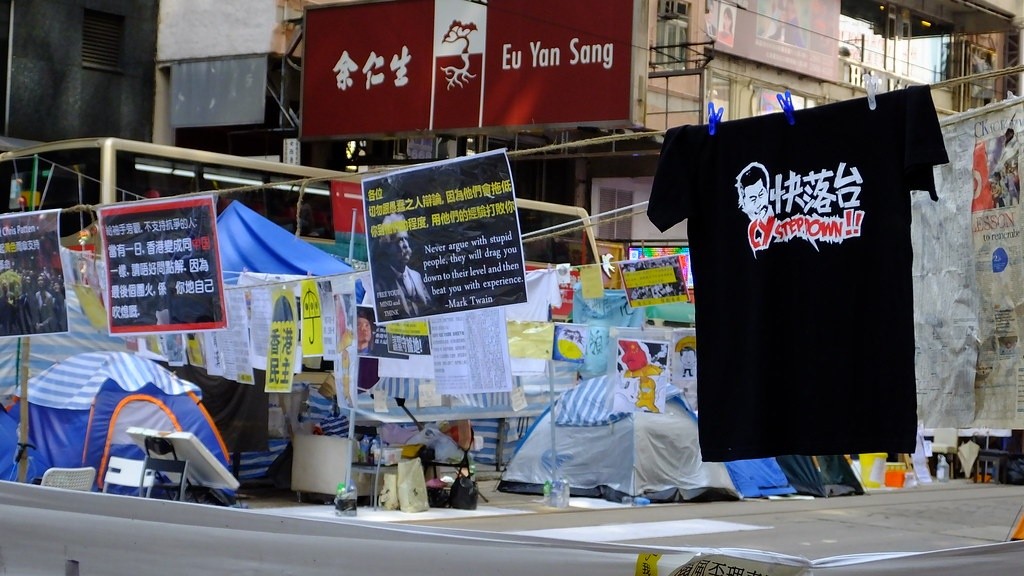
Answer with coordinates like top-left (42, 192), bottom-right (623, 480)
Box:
top-left (970, 450), bottom-right (1008, 483)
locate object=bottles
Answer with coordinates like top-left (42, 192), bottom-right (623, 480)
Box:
top-left (936, 457), bottom-right (949, 482)
top-left (336, 483), bottom-right (346, 517)
top-left (346, 479), bottom-right (357, 516)
top-left (544, 476), bottom-right (569, 508)
top-left (622, 497), bottom-right (650, 505)
top-left (361, 435), bottom-right (369, 463)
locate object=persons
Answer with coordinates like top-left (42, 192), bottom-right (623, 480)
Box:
top-left (703, 0), bottom-right (718, 37)
top-left (0, 260), bottom-right (68, 337)
top-left (989, 156), bottom-right (1020, 209)
top-left (372, 211), bottom-right (434, 322)
top-left (356, 315), bottom-right (373, 355)
top-left (719, 7), bottom-right (735, 46)
top-left (621, 256), bottom-right (687, 299)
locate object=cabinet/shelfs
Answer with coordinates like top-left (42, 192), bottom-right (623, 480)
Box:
top-left (290, 433), bottom-right (396, 503)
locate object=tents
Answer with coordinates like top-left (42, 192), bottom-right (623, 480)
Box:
top-left (493, 373), bottom-right (744, 506)
top-left (5, 351), bottom-right (238, 511)
top-left (216, 200), bottom-right (577, 491)
top-left (723, 456), bottom-right (797, 498)
top-left (1, 403), bottom-right (51, 489)
top-left (0, 244), bottom-right (127, 402)
top-left (776, 454), bottom-right (869, 498)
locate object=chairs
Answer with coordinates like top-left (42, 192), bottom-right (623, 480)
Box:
top-left (930, 428), bottom-right (957, 481)
top-left (39, 455), bottom-right (187, 501)
top-left (429, 426), bottom-right (490, 507)
top-left (352, 419), bottom-right (395, 511)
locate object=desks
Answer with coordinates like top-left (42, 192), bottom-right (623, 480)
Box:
top-left (350, 403), bottom-right (550, 474)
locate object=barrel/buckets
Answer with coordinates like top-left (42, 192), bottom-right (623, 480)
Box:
top-left (858, 453), bottom-right (888, 488)
top-left (885, 462), bottom-right (905, 489)
